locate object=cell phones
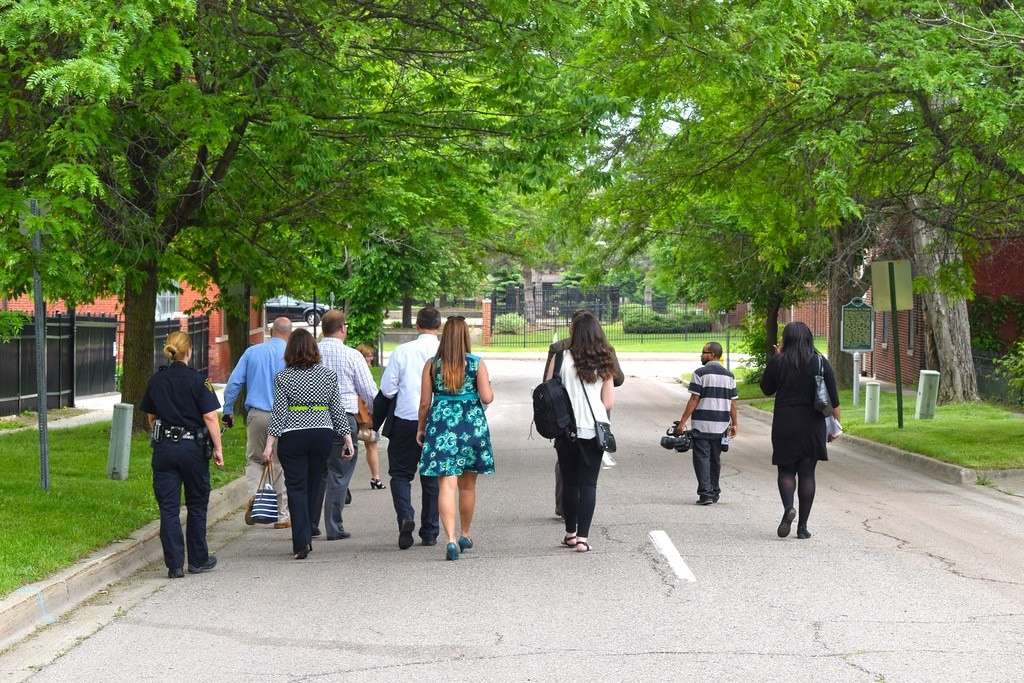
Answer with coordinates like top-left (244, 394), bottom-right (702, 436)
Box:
top-left (221, 414), bottom-right (233, 428)
top-left (420, 434), bottom-right (425, 443)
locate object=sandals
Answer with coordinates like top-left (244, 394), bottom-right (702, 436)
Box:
top-left (575, 541), bottom-right (592, 551)
top-left (561, 536), bottom-right (576, 548)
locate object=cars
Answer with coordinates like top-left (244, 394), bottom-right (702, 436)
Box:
top-left (267, 295), bottom-right (329, 326)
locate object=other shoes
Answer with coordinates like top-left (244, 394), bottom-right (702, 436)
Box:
top-left (312, 529), bottom-right (321, 536)
top-left (328, 532), bottom-right (350, 540)
top-left (778, 508), bottom-right (796, 537)
top-left (344, 489), bottom-right (352, 504)
top-left (274, 519), bottom-right (291, 529)
top-left (695, 496), bottom-right (713, 504)
top-left (398, 520), bottom-right (416, 550)
top-left (422, 539), bottom-right (437, 546)
top-left (245, 495), bottom-right (257, 525)
top-left (797, 530), bottom-right (812, 539)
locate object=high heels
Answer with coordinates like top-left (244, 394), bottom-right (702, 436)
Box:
top-left (459, 536), bottom-right (473, 552)
top-left (371, 478), bottom-right (386, 489)
top-left (446, 542), bottom-right (459, 561)
top-left (294, 538), bottom-right (313, 559)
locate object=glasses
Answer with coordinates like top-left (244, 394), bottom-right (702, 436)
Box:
top-left (702, 351), bottom-right (712, 354)
top-left (340, 323), bottom-right (349, 328)
top-left (447, 316), bottom-right (465, 320)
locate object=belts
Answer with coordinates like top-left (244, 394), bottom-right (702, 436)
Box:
top-left (161, 429), bottom-right (195, 439)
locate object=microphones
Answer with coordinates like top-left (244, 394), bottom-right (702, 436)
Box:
top-left (720, 429), bottom-right (729, 452)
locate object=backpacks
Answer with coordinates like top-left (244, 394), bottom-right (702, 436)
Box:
top-left (528, 351), bottom-right (577, 442)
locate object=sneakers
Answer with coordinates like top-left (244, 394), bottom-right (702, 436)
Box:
top-left (188, 557), bottom-right (217, 573)
top-left (168, 568), bottom-right (184, 578)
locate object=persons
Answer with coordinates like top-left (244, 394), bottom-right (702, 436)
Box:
top-left (379, 306), bottom-right (449, 548)
top-left (675, 342), bottom-right (738, 506)
top-left (417, 316), bottom-right (494, 561)
top-left (315, 332), bottom-right (352, 505)
top-left (759, 322), bottom-right (841, 538)
top-left (139, 331), bottom-right (225, 580)
top-left (312, 309), bottom-right (379, 541)
top-left (352, 343), bottom-right (386, 490)
top-left (263, 328), bottom-right (356, 559)
top-left (543, 310), bottom-right (623, 519)
top-left (546, 312), bottom-right (615, 552)
top-left (222, 317), bottom-right (296, 529)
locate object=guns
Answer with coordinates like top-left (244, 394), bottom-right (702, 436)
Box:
top-left (203, 427), bottom-right (213, 459)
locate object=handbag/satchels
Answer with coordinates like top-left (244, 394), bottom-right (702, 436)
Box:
top-left (251, 463), bottom-right (278, 524)
top-left (814, 353), bottom-right (833, 418)
top-left (594, 421), bottom-right (616, 453)
